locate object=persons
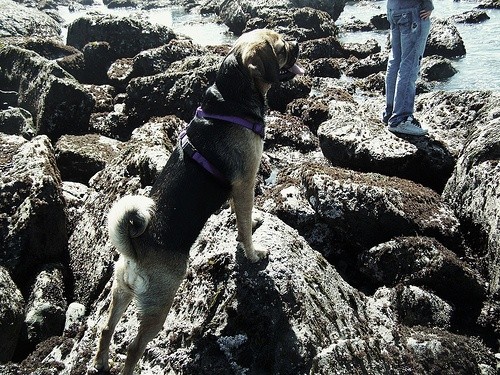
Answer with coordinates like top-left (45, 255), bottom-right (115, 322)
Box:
top-left (381, 0), bottom-right (436, 135)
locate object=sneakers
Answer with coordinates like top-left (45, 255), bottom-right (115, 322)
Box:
top-left (386, 120), bottom-right (428, 135)
top-left (384, 115), bottom-right (414, 125)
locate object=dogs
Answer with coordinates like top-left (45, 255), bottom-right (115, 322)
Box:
top-left (93, 28), bottom-right (305, 375)
